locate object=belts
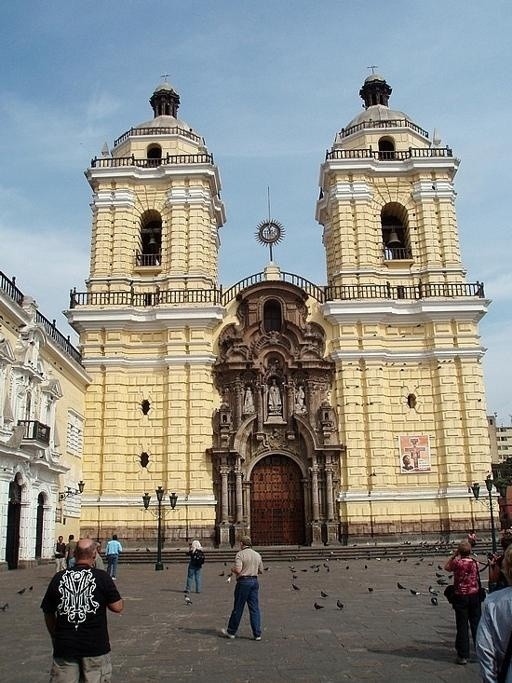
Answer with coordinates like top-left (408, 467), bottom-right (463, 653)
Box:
top-left (238, 575), bottom-right (258, 580)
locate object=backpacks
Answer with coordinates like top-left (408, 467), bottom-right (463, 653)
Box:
top-left (190, 548), bottom-right (206, 569)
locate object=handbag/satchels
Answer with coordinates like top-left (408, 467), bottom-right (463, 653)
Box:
top-left (478, 586), bottom-right (486, 603)
top-left (442, 584), bottom-right (465, 605)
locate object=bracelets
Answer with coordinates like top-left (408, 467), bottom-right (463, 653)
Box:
top-left (451, 555), bottom-right (454, 559)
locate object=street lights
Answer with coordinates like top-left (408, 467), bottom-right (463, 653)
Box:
top-left (471, 475), bottom-right (506, 554)
top-left (143, 486), bottom-right (178, 570)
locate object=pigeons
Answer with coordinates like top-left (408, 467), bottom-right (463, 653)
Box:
top-left (321, 591), bottom-right (328, 598)
top-left (219, 571), bottom-right (224, 576)
top-left (337, 600), bottom-right (344, 610)
top-left (224, 573), bottom-right (233, 583)
top-left (185, 595), bottom-right (192, 605)
top-left (314, 602), bottom-right (324, 611)
top-left (0, 604), bottom-right (8, 612)
top-left (18, 589), bottom-right (25, 595)
top-left (288, 537), bottom-right (500, 596)
top-left (431, 598), bottom-right (438, 606)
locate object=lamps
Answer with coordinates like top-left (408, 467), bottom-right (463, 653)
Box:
top-left (59, 481), bottom-right (84, 501)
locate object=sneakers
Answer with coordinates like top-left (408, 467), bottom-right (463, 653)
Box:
top-left (220, 627), bottom-right (235, 639)
top-left (111, 576), bottom-right (117, 580)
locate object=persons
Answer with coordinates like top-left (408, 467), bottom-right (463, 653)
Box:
top-left (39, 538), bottom-right (124, 682)
top-left (185, 539), bottom-right (205, 592)
top-left (444, 540), bottom-right (483, 665)
top-left (295, 386), bottom-right (305, 405)
top-left (54, 535), bottom-right (67, 572)
top-left (269, 382), bottom-right (281, 405)
top-left (95, 541), bottom-right (105, 571)
top-left (220, 535), bottom-right (264, 641)
top-left (106, 534), bottom-right (123, 580)
top-left (475, 543), bottom-right (511, 683)
top-left (244, 386), bottom-right (254, 406)
top-left (65, 534), bottom-right (77, 568)
top-left (466, 529), bottom-right (480, 547)
top-left (488, 529), bottom-right (511, 594)
